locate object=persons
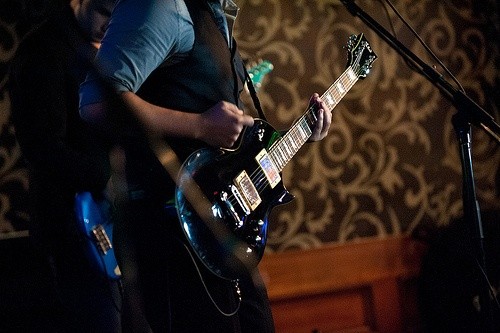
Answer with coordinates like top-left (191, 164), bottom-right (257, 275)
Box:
top-left (76, 0), bottom-right (335, 333)
top-left (5, 1), bottom-right (156, 333)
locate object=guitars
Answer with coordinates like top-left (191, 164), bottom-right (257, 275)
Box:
top-left (75, 60), bottom-right (275, 284)
top-left (172, 31), bottom-right (379, 284)
